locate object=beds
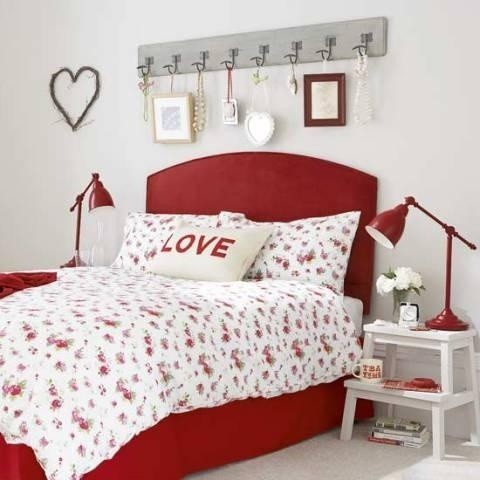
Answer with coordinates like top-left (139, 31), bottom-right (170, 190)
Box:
top-left (1, 151), bottom-right (380, 480)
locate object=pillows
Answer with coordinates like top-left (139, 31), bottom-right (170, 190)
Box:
top-left (111, 208), bottom-right (245, 286)
top-left (221, 212), bottom-right (360, 295)
top-left (149, 219), bottom-right (275, 282)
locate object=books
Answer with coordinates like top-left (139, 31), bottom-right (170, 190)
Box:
top-left (367, 416), bottom-right (430, 450)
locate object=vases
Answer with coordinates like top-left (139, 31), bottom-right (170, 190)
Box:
top-left (390, 287), bottom-right (410, 326)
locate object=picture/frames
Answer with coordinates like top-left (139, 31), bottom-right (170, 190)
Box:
top-left (245, 110), bottom-right (276, 147)
top-left (148, 92), bottom-right (196, 146)
top-left (302, 71), bottom-right (347, 129)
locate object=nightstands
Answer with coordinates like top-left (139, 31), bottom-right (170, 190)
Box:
top-left (337, 319), bottom-right (480, 462)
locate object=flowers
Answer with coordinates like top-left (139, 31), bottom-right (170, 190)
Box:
top-left (374, 267), bottom-right (426, 297)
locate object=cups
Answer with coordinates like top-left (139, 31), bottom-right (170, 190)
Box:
top-left (352, 358), bottom-right (384, 386)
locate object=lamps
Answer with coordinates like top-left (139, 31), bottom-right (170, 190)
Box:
top-left (366, 192), bottom-right (479, 331)
top-left (59, 170), bottom-right (116, 269)
